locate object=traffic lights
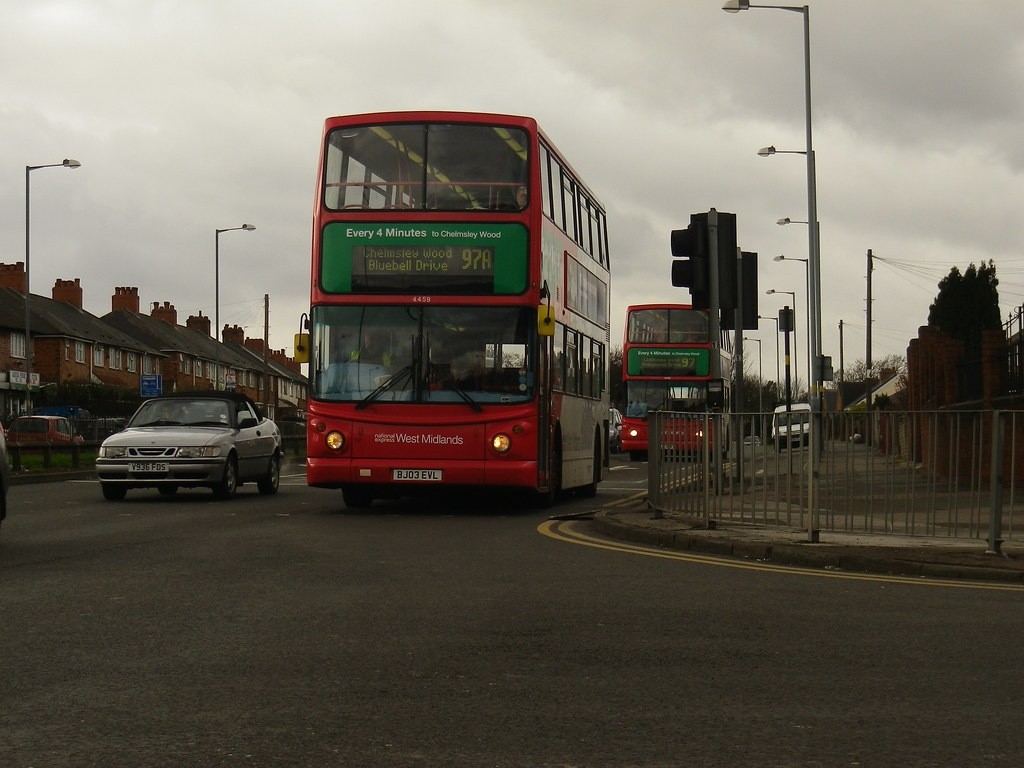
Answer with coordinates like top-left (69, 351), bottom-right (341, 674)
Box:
top-left (671, 212), bottom-right (708, 309)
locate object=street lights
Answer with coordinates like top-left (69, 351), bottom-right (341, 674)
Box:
top-left (743, 337), bottom-right (763, 446)
top-left (773, 254), bottom-right (810, 404)
top-left (755, 146), bottom-right (817, 223)
top-left (25, 159), bottom-right (84, 418)
top-left (216, 224), bottom-right (258, 390)
top-left (765, 289), bottom-right (799, 404)
top-left (722, 0), bottom-right (820, 481)
top-left (775, 217), bottom-right (823, 357)
top-left (758, 315), bottom-right (780, 404)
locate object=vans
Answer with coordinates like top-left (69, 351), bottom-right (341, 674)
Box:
top-left (771, 403), bottom-right (811, 454)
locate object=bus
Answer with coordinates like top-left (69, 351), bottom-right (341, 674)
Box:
top-left (292, 110), bottom-right (615, 511)
top-left (621, 303), bottom-right (735, 463)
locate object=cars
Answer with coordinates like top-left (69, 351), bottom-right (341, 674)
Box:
top-left (743, 436), bottom-right (762, 447)
top-left (95, 390), bottom-right (284, 501)
top-left (609, 408), bottom-right (623, 450)
top-left (7, 416), bottom-right (85, 446)
top-left (0, 422), bottom-right (9, 520)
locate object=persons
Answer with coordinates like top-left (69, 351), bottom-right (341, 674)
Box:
top-left (162, 403), bottom-right (175, 421)
top-left (442, 358), bottom-right (478, 391)
top-left (0, 422), bottom-right (8, 526)
top-left (360, 331), bottom-right (388, 366)
